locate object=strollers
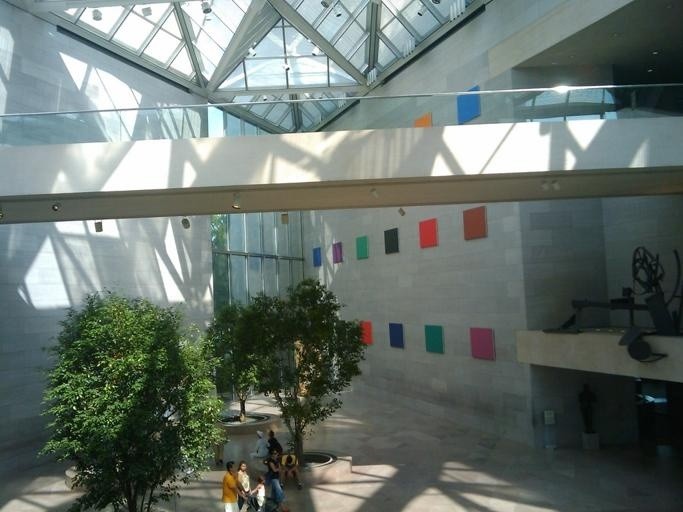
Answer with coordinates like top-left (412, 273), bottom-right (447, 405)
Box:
top-left (246, 493), bottom-right (281, 512)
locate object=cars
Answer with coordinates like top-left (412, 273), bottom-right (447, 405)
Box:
top-left (645, 394), bottom-right (667, 403)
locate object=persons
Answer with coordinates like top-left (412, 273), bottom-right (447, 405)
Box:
top-left (213, 428), bottom-right (305, 512)
top-left (578, 381), bottom-right (597, 434)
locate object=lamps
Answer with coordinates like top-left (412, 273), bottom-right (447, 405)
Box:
top-left (92, 9), bottom-right (103, 22)
top-left (279, 213), bottom-right (290, 225)
top-left (180, 218), bottom-right (191, 230)
top-left (416, 4), bottom-right (428, 18)
top-left (141, 7), bottom-right (154, 18)
top-left (318, 2), bottom-right (344, 18)
top-left (94, 221), bottom-right (104, 233)
top-left (200, 1), bottom-right (214, 15)
top-left (369, 188), bottom-right (379, 199)
top-left (234, 38), bottom-right (330, 106)
top-left (397, 206), bottom-right (407, 218)
